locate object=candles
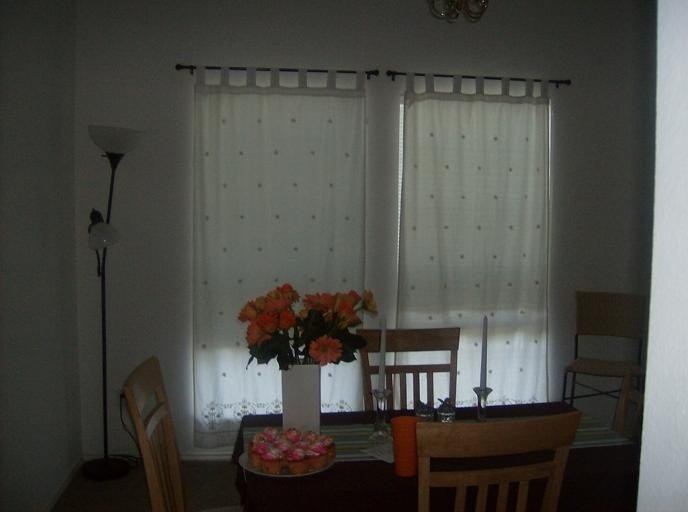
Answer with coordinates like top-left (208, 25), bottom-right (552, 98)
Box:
top-left (479, 314), bottom-right (490, 390)
top-left (377, 314), bottom-right (388, 393)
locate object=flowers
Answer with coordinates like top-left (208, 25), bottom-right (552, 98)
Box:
top-left (230, 279), bottom-right (382, 374)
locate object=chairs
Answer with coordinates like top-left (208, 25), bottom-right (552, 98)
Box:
top-left (351, 320), bottom-right (462, 418)
top-left (558, 286), bottom-right (649, 441)
top-left (118, 353), bottom-right (245, 511)
top-left (410, 403), bottom-right (581, 512)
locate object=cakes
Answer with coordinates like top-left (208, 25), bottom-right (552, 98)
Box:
top-left (249, 425), bottom-right (337, 475)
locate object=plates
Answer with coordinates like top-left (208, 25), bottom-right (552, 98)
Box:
top-left (236, 442), bottom-right (336, 480)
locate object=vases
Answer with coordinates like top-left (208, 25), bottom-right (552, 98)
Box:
top-left (277, 355), bottom-right (323, 438)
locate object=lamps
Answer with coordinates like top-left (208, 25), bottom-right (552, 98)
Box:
top-left (72, 122), bottom-right (147, 487)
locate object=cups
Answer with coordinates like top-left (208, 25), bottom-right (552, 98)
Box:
top-left (391, 415), bottom-right (423, 480)
